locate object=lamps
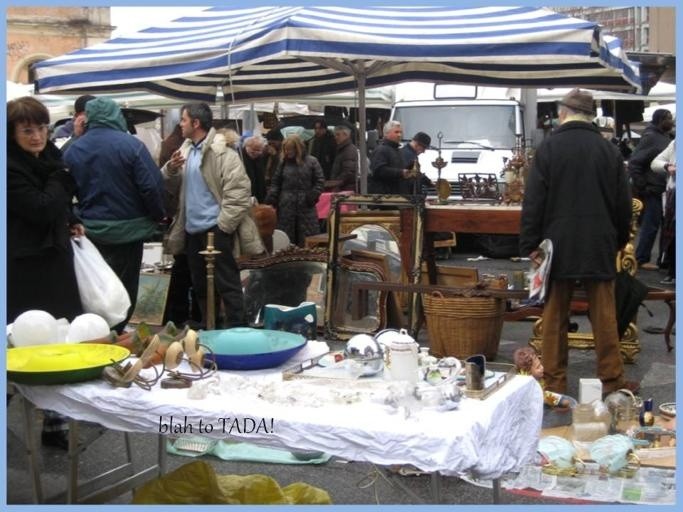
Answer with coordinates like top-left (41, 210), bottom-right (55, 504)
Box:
top-left (161, 331), bottom-right (218, 389)
top-left (100, 334), bottom-right (166, 391)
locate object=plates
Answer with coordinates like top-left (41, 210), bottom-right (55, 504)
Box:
top-left (195, 328), bottom-right (306, 371)
top-left (659, 403), bottom-right (676, 421)
top-left (6, 330), bottom-right (131, 385)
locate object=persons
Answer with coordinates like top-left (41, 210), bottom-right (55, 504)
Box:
top-left (645, 134), bottom-right (675, 284)
top-left (5, 96), bottom-right (92, 454)
top-left (513, 349), bottom-right (581, 411)
top-left (154, 99), bottom-right (440, 330)
top-left (630, 109), bottom-right (675, 270)
top-left (59, 97), bottom-right (169, 340)
top-left (517, 88), bottom-right (641, 404)
top-left (51, 92), bottom-right (96, 151)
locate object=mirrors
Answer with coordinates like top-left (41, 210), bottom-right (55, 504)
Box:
top-left (334, 210), bottom-right (411, 313)
top-left (220, 243), bottom-right (389, 340)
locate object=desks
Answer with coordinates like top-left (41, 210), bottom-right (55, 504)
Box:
top-left (416, 197), bottom-right (524, 235)
top-left (645, 285), bottom-right (676, 353)
top-left (7, 334), bottom-right (544, 505)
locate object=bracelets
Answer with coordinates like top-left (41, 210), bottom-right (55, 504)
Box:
top-left (663, 162), bottom-right (672, 173)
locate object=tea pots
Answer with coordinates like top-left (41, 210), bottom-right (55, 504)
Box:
top-left (390, 328), bottom-right (420, 372)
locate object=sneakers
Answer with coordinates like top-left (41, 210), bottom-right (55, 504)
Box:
top-left (640, 262), bottom-right (659, 271)
top-left (621, 380), bottom-right (641, 395)
top-left (40, 429), bottom-right (68, 452)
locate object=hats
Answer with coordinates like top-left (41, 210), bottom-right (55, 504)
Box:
top-left (555, 86), bottom-right (596, 115)
top-left (73, 94), bottom-right (98, 111)
top-left (411, 131), bottom-right (431, 150)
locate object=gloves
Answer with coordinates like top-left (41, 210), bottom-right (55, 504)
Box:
top-left (421, 291), bottom-right (507, 364)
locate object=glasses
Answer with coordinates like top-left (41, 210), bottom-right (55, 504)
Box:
top-left (16, 124), bottom-right (50, 136)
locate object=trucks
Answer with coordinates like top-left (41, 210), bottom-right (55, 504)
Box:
top-left (386, 75), bottom-right (539, 256)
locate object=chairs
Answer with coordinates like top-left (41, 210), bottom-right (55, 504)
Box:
top-left (528, 198), bottom-right (643, 365)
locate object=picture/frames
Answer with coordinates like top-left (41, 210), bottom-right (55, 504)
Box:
top-left (415, 262), bottom-right (478, 287)
top-left (325, 193), bottom-right (425, 342)
top-left (126, 271), bottom-right (172, 327)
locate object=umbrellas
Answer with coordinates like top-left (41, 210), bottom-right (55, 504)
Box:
top-left (25, 3), bottom-right (645, 209)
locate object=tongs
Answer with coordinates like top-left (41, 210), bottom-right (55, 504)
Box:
top-left (282, 351), bottom-right (342, 381)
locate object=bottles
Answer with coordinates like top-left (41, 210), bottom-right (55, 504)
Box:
top-left (419, 347), bottom-right (429, 365)
top-left (544, 391), bottom-right (570, 407)
top-left (426, 365), bottom-right (441, 384)
top-left (640, 400), bottom-right (654, 426)
